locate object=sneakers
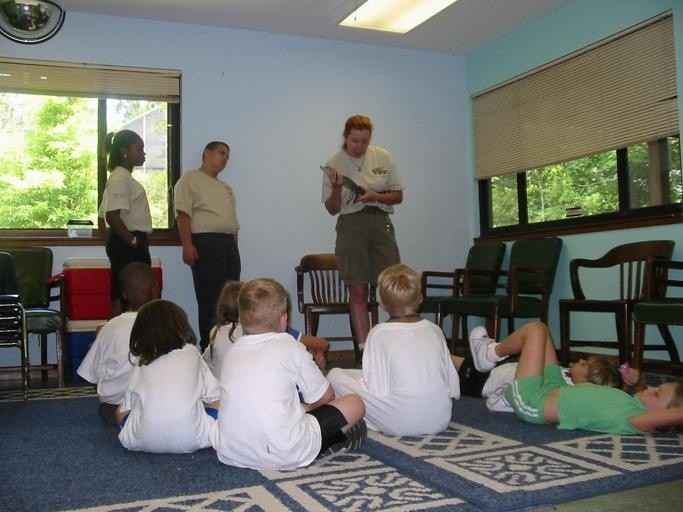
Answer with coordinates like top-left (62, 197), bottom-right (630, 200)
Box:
top-left (313, 417), bottom-right (370, 461)
top-left (469, 325), bottom-right (498, 373)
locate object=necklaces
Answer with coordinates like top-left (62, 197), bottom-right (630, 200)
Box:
top-left (389, 313), bottom-right (419, 320)
top-left (348, 154), bottom-right (366, 171)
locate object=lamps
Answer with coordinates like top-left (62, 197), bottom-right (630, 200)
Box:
top-left (0, 1), bottom-right (65, 47)
top-left (329, 0), bottom-right (468, 38)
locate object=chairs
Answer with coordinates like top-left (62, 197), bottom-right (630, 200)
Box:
top-left (0, 250), bottom-right (33, 403)
top-left (0, 247), bottom-right (66, 385)
top-left (291, 235), bottom-right (563, 365)
top-left (556, 235), bottom-right (680, 376)
top-left (621, 252), bottom-right (683, 379)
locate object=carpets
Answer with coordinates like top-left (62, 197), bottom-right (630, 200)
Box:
top-left (0, 356), bottom-right (683, 511)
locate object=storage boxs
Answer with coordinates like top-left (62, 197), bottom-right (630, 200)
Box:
top-left (57, 251), bottom-right (164, 322)
top-left (49, 315), bottom-right (110, 387)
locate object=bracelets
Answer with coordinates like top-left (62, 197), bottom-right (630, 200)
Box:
top-left (131, 237), bottom-right (136, 248)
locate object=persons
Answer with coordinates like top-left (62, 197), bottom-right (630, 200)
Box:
top-left (326, 265), bottom-right (463, 436)
top-left (75, 262), bottom-right (157, 427)
top-left (468, 322), bottom-right (681, 434)
top-left (97, 129), bottom-right (153, 320)
top-left (449, 352), bottom-right (647, 412)
top-left (319, 115), bottom-right (406, 357)
top-left (174, 141), bottom-right (240, 352)
top-left (207, 278), bottom-right (367, 469)
top-left (202, 278), bottom-right (246, 378)
top-left (283, 296), bottom-right (327, 372)
top-left (111, 298), bottom-right (218, 453)
